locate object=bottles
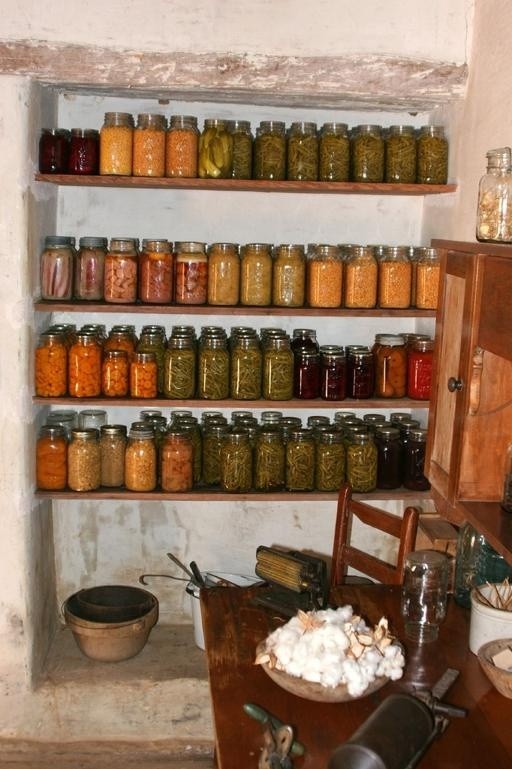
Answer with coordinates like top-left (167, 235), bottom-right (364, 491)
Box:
top-left (401, 549), bottom-right (451, 643)
top-left (500, 442), bottom-right (512, 514)
top-left (452, 521), bottom-right (487, 609)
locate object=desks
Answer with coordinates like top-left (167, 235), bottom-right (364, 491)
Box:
top-left (199, 582), bottom-right (512, 769)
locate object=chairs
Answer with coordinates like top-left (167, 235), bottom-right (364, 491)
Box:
top-left (332, 488), bottom-right (419, 585)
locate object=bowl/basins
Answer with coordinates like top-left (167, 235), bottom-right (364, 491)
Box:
top-left (476, 638), bottom-right (512, 701)
top-left (254, 622), bottom-right (405, 703)
top-left (64, 583), bottom-right (159, 663)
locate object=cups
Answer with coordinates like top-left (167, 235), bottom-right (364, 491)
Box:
top-left (465, 582), bottom-right (512, 661)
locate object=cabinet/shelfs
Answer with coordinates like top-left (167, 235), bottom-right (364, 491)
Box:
top-left (421, 239), bottom-right (512, 563)
top-left (33, 174), bottom-right (457, 504)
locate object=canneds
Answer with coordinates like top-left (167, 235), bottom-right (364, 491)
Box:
top-left (34, 411), bottom-right (427, 492)
top-left (33, 324), bottom-right (433, 397)
top-left (32, 111), bottom-right (451, 182)
top-left (41, 236), bottom-right (440, 309)
